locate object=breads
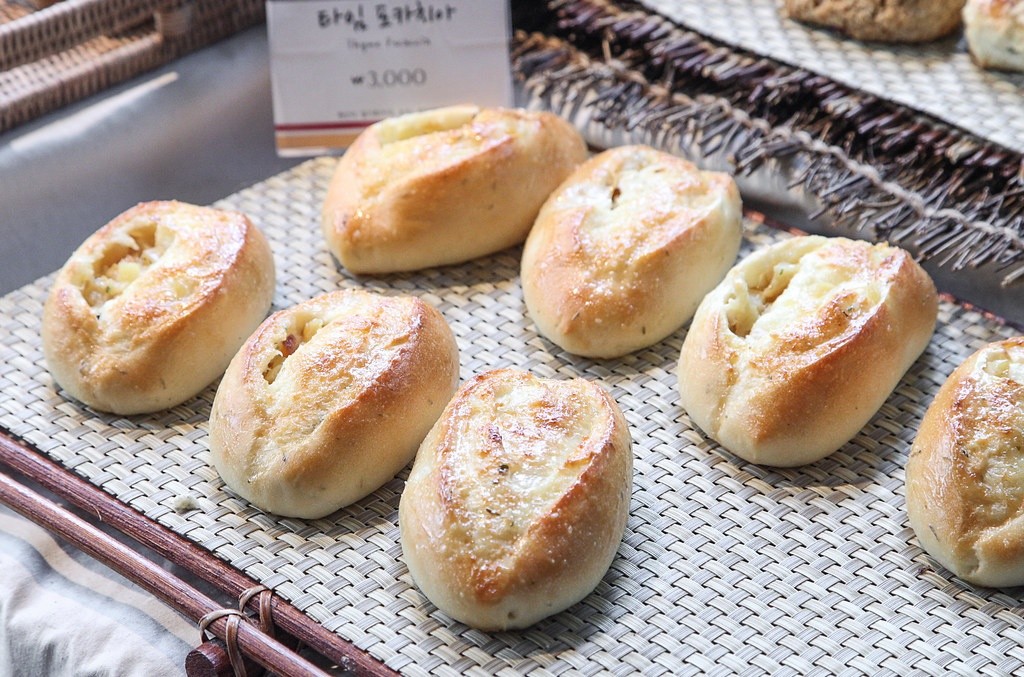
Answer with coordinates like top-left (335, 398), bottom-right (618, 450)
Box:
top-left (785, 0), bottom-right (1024, 74)
top-left (321, 106), bottom-right (591, 275)
top-left (207, 288), bottom-right (459, 520)
top-left (905, 335), bottom-right (1024, 588)
top-left (521, 143), bottom-right (742, 356)
top-left (676, 233), bottom-right (939, 467)
top-left (41, 198), bottom-right (275, 415)
top-left (397, 368), bottom-right (635, 629)
